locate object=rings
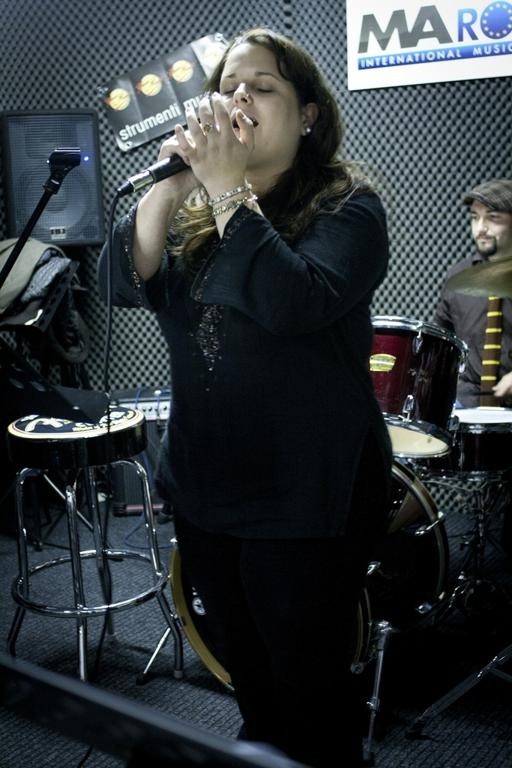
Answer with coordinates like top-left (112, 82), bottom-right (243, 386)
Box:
top-left (202, 122), bottom-right (212, 135)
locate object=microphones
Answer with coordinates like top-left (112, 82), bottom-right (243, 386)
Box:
top-left (116, 153), bottom-right (190, 198)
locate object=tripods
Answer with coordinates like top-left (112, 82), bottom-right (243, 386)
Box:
top-left (437, 479), bottom-right (512, 621)
top-left (31, 245), bottom-right (122, 553)
top-left (402, 641), bottom-right (511, 740)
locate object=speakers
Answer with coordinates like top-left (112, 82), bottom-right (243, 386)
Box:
top-left (0, 108), bottom-right (107, 246)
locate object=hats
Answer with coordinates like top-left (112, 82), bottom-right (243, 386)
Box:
top-left (464, 180), bottom-right (512, 212)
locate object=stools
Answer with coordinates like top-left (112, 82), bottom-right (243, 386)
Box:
top-left (6, 407), bottom-right (185, 683)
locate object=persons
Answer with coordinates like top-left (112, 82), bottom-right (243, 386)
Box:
top-left (435, 177), bottom-right (512, 406)
top-left (93, 26), bottom-right (391, 765)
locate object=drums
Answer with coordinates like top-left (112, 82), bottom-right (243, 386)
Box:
top-left (368, 317), bottom-right (467, 458)
top-left (171, 462), bottom-right (448, 709)
top-left (433, 405), bottom-right (512, 481)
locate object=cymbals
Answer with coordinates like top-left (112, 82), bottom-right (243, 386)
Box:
top-left (446, 257), bottom-right (511, 298)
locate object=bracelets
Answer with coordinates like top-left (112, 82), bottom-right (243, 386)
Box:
top-left (212, 194), bottom-right (257, 219)
top-left (209, 183), bottom-right (252, 208)
